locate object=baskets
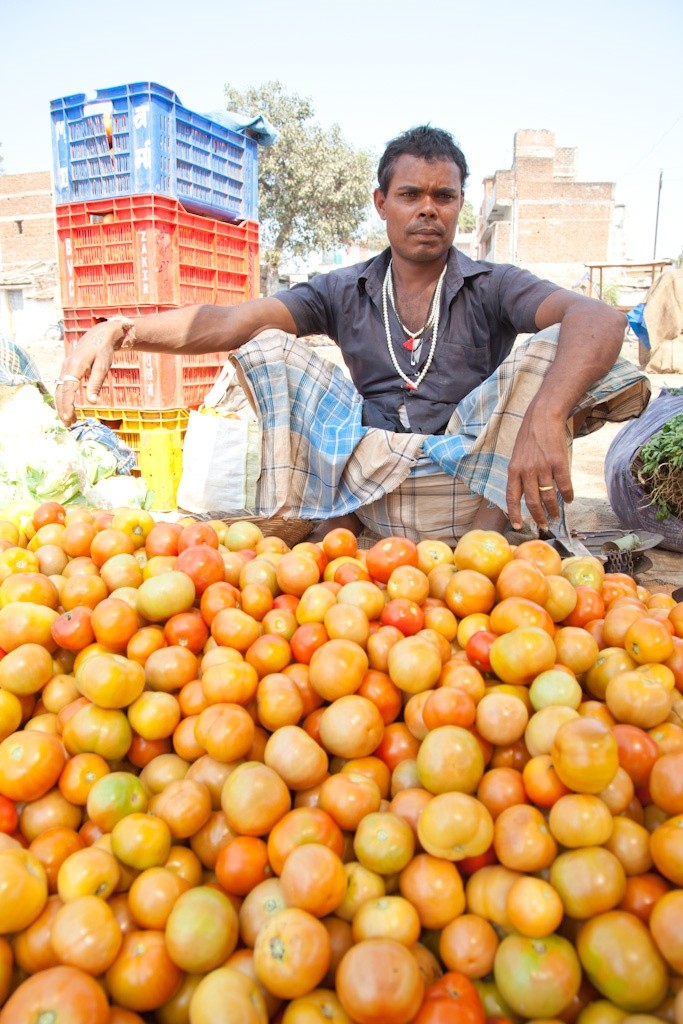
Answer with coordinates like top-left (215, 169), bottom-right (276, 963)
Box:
top-left (220, 514), bottom-right (313, 549)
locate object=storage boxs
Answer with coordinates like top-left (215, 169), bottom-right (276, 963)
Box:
top-left (51, 80), bottom-right (262, 514)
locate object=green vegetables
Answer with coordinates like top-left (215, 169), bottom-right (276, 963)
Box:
top-left (630, 380), bottom-right (683, 521)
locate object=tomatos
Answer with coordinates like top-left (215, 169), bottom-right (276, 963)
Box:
top-left (0, 501), bottom-right (683, 1024)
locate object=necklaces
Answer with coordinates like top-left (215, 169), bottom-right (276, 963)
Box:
top-left (383, 256), bottom-right (448, 390)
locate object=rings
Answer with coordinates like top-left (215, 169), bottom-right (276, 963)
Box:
top-left (539, 486), bottom-right (553, 492)
top-left (56, 374), bottom-right (81, 389)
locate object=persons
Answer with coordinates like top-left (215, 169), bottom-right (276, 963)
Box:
top-left (55, 122), bottom-right (623, 543)
